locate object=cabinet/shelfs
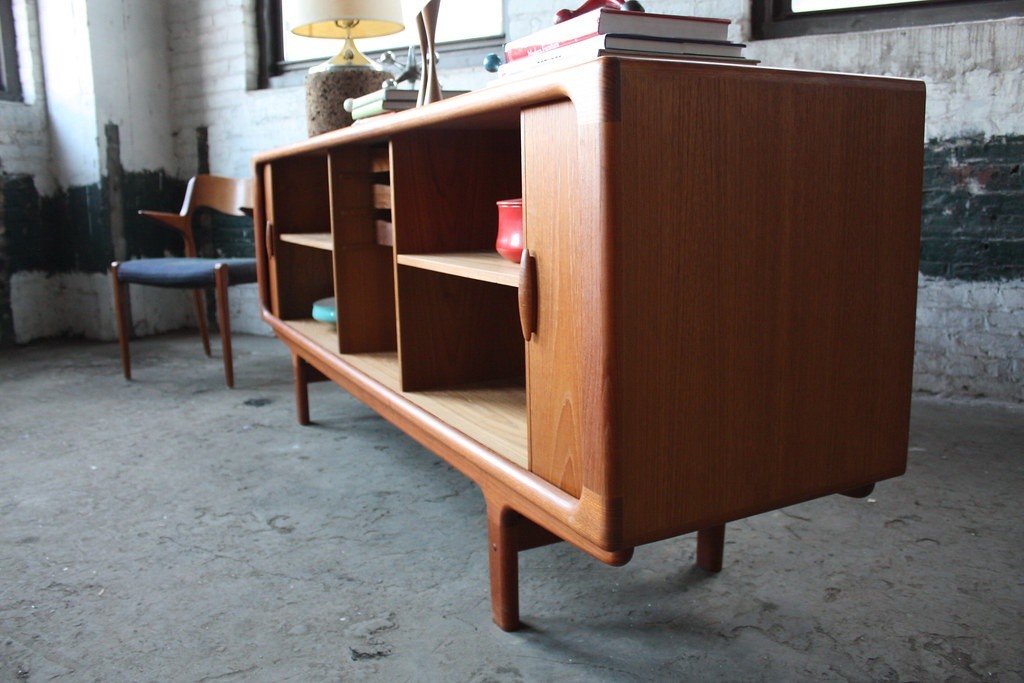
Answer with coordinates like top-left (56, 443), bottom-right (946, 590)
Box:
top-left (251, 53), bottom-right (926, 633)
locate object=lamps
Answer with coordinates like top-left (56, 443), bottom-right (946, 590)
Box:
top-left (286, 0), bottom-right (406, 138)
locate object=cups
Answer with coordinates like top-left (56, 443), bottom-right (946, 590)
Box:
top-left (495, 198), bottom-right (523, 264)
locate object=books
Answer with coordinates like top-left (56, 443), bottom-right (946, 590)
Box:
top-left (494, 5), bottom-right (763, 86)
top-left (349, 88), bottom-right (475, 121)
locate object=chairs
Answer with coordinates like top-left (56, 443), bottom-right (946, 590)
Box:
top-left (111, 175), bottom-right (256, 388)
